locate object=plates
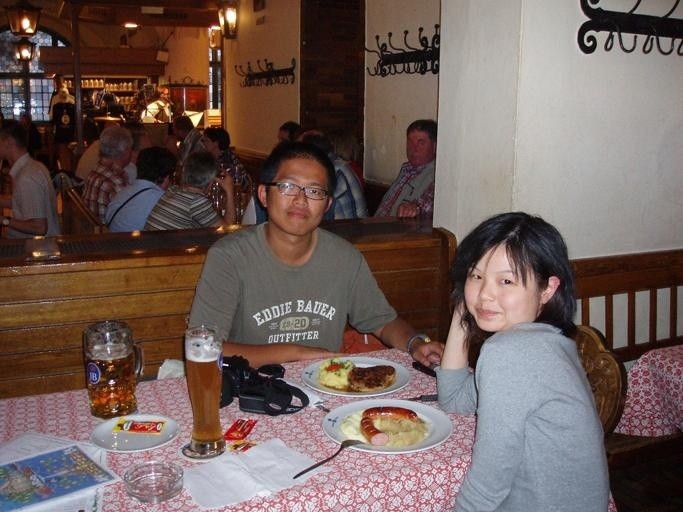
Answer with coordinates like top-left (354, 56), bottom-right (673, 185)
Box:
top-left (300, 356), bottom-right (411, 397)
top-left (90, 412), bottom-right (181, 454)
top-left (322, 399), bottom-right (453, 454)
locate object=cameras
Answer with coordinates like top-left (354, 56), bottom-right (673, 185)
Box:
top-left (220, 355), bottom-right (292, 416)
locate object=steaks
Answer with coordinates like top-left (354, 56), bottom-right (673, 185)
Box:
top-left (348, 366), bottom-right (395, 392)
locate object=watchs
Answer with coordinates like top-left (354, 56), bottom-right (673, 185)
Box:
top-left (406, 333), bottom-right (432, 354)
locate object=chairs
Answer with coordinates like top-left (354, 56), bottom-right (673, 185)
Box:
top-left (571, 322), bottom-right (627, 438)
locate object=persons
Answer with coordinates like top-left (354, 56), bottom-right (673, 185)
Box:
top-left (373, 119), bottom-right (437, 219)
top-left (0, 112), bottom-right (59, 241)
top-left (187, 140), bottom-right (446, 368)
top-left (53, 73), bottom-right (257, 234)
top-left (243, 121), bottom-right (370, 226)
top-left (432, 211), bottom-right (610, 512)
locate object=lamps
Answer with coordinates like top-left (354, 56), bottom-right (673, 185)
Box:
top-left (10, 36), bottom-right (37, 65)
top-left (217, 5), bottom-right (237, 39)
top-left (1, 0), bottom-right (43, 38)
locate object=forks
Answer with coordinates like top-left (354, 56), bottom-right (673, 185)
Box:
top-left (292, 438), bottom-right (362, 479)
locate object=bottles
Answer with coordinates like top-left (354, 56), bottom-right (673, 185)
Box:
top-left (62, 79), bottom-right (134, 113)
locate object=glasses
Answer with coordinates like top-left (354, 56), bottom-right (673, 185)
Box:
top-left (258, 180), bottom-right (331, 201)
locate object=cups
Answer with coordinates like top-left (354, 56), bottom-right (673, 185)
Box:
top-left (184, 325), bottom-right (225, 455)
top-left (206, 154), bottom-right (249, 223)
top-left (82, 320), bottom-right (142, 422)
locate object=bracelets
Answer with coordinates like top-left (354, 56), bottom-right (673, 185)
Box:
top-left (416, 205), bottom-right (423, 215)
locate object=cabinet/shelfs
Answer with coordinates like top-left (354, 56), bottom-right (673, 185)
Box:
top-left (63, 75), bottom-right (152, 115)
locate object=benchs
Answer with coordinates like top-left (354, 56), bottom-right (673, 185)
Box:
top-left (0, 123), bottom-right (256, 237)
top-left (558, 248), bottom-right (683, 471)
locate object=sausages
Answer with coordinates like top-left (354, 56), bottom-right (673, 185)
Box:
top-left (363, 407), bottom-right (417, 422)
top-left (360, 417), bottom-right (387, 445)
